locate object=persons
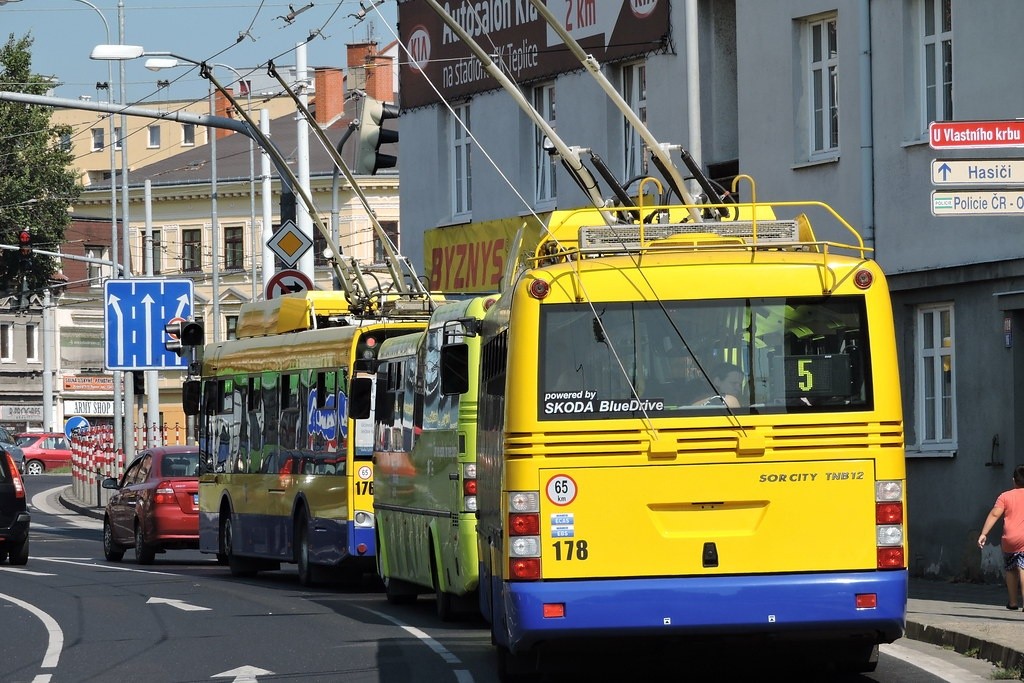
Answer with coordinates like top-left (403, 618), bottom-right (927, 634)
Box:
top-left (689, 364), bottom-right (744, 405)
top-left (977, 465), bottom-right (1024, 611)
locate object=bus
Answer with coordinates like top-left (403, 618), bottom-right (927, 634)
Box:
top-left (440, 173), bottom-right (910, 682)
top-left (183, 290), bottom-right (445, 593)
top-left (348, 293), bottom-right (503, 622)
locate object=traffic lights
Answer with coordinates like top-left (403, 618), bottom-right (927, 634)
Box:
top-left (355, 96), bottom-right (399, 176)
top-left (165, 323), bottom-right (185, 357)
top-left (181, 322), bottom-right (204, 347)
top-left (19, 231), bottom-right (34, 273)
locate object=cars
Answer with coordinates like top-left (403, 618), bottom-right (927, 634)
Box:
top-left (0, 427), bottom-right (26, 475)
top-left (254, 450), bottom-right (302, 474)
top-left (102, 446), bottom-right (200, 566)
top-left (15, 433), bottom-right (72, 475)
top-left (0, 446), bottom-right (31, 565)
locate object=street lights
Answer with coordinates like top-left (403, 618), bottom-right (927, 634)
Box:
top-left (0, 0), bottom-right (118, 279)
top-left (144, 58), bottom-right (257, 304)
top-left (89, 45), bottom-right (220, 343)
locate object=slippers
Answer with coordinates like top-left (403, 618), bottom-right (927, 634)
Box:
top-left (1006, 602), bottom-right (1019, 610)
